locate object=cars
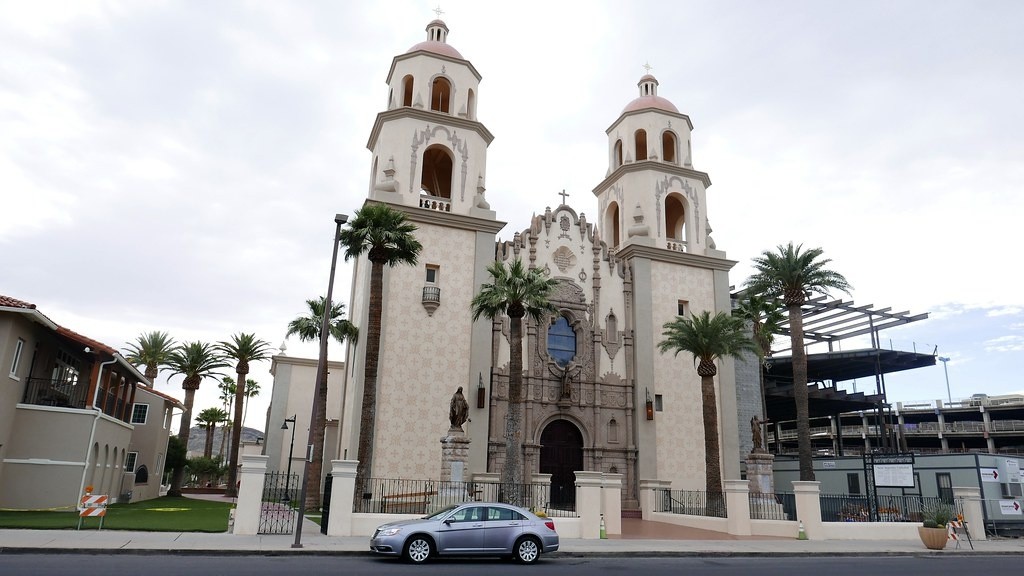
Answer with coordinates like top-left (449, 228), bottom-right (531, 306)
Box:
top-left (370, 501), bottom-right (561, 564)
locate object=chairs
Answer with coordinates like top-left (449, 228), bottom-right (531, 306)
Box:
top-left (476, 508), bottom-right (482, 520)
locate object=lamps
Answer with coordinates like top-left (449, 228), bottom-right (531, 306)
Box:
top-left (477, 372), bottom-right (485, 408)
top-left (83, 347), bottom-right (95, 353)
top-left (645, 387), bottom-right (654, 421)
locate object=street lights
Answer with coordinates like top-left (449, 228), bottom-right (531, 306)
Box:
top-left (290, 213), bottom-right (350, 554)
top-left (938, 356), bottom-right (953, 410)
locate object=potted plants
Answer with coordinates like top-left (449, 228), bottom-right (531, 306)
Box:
top-left (917, 507), bottom-right (956, 550)
